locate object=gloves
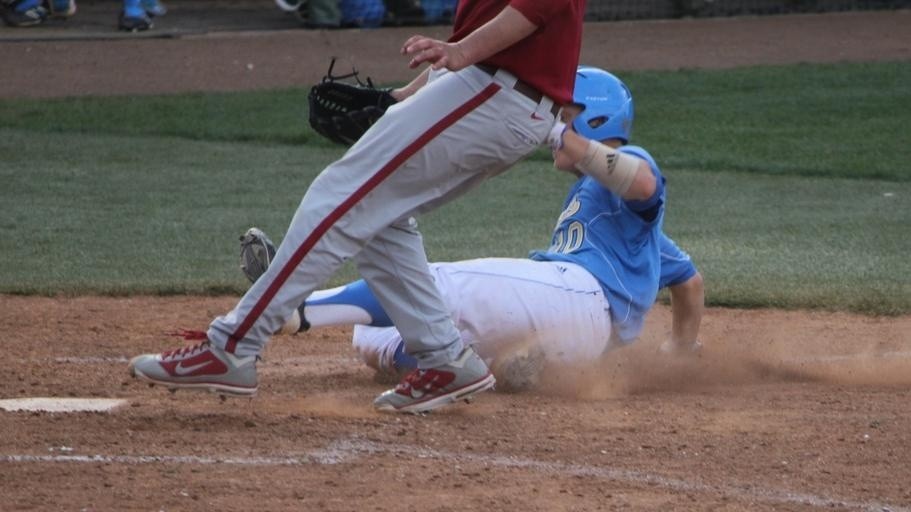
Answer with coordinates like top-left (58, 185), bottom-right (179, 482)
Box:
top-left (542, 119), bottom-right (567, 153)
top-left (660, 338), bottom-right (705, 357)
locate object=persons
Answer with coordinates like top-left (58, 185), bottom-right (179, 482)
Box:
top-left (119, 0), bottom-right (167, 33)
top-left (238, 65), bottom-right (706, 390)
top-left (126, 0), bottom-right (586, 414)
top-left (1, 0), bottom-right (76, 27)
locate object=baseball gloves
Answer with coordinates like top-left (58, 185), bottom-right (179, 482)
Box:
top-left (308, 82), bottom-right (398, 145)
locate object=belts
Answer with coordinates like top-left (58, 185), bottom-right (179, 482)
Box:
top-left (473, 60), bottom-right (563, 119)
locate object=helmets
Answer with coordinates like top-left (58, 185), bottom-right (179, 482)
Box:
top-left (570, 64), bottom-right (636, 145)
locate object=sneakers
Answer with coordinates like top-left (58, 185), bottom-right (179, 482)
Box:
top-left (127, 341), bottom-right (260, 398)
top-left (237, 225), bottom-right (313, 336)
top-left (372, 348), bottom-right (500, 415)
